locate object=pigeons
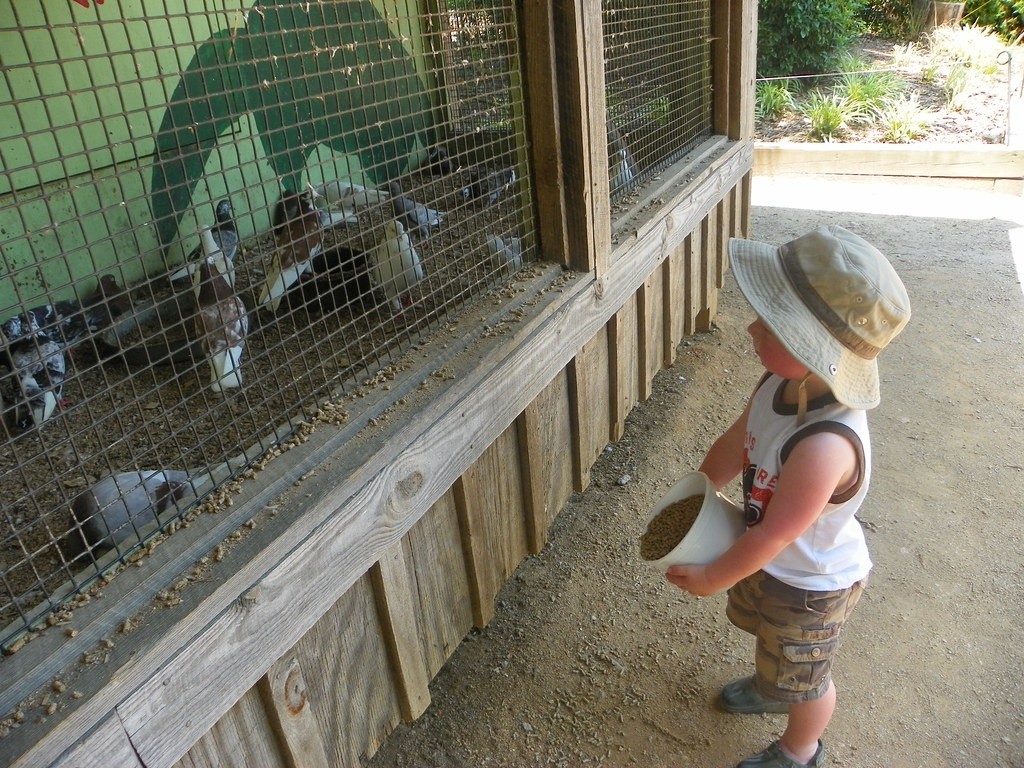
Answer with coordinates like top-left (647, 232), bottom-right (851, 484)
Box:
top-left (0, 126), bottom-right (634, 567)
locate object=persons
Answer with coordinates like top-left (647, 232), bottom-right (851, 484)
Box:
top-left (666, 224), bottom-right (912, 767)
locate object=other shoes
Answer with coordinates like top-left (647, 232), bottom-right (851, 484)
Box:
top-left (736, 738), bottom-right (825, 768)
top-left (719, 676), bottom-right (792, 715)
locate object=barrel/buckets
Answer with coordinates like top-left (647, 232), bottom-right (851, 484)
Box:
top-left (639, 471), bottom-right (747, 577)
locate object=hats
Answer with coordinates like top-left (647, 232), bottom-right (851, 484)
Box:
top-left (729, 223), bottom-right (912, 410)
